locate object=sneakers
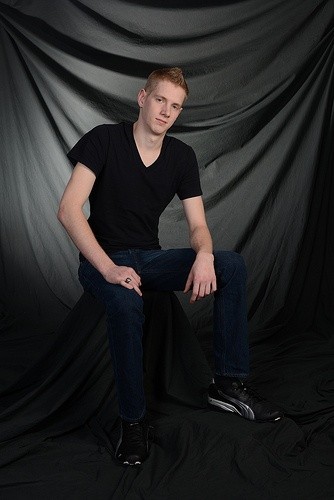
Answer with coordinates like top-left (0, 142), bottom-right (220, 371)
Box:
top-left (210, 377), bottom-right (278, 423)
top-left (113, 420), bottom-right (152, 465)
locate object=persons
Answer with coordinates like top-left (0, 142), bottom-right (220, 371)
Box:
top-left (56, 63), bottom-right (263, 468)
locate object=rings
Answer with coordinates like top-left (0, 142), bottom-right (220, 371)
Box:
top-left (125, 278), bottom-right (131, 283)
